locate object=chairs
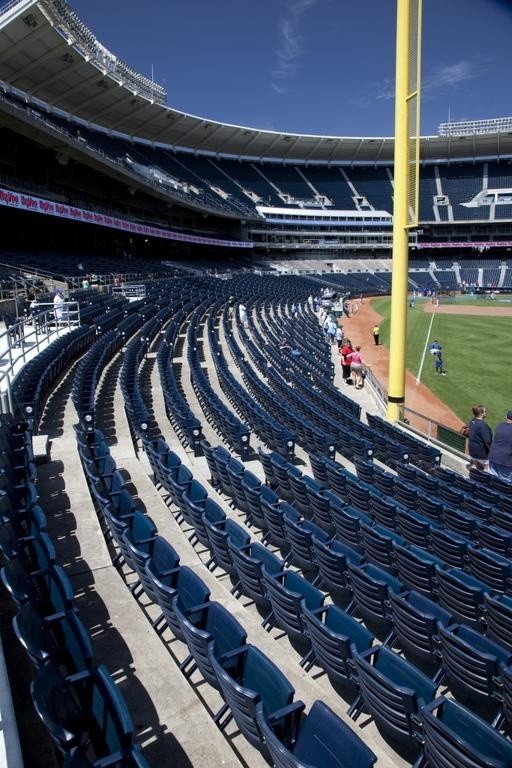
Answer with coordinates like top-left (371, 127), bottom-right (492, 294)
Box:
top-left (0, 255), bottom-right (347, 400)
top-left (347, 255), bottom-right (512, 299)
top-left (1, 399), bottom-right (512, 768)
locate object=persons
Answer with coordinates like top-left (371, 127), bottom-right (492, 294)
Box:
top-left (429, 340), bottom-right (446, 376)
top-left (228, 296), bottom-right (235, 321)
top-left (308, 287), bottom-right (372, 389)
top-left (468, 404), bottom-right (512, 485)
top-left (239, 301), bottom-right (249, 329)
top-left (82, 272), bottom-right (121, 289)
top-left (373, 325), bottom-right (380, 345)
top-left (25, 287), bottom-right (64, 327)
top-left (408, 295), bottom-right (416, 311)
top-left (278, 337), bottom-right (302, 357)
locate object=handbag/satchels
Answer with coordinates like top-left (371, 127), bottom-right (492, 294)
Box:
top-left (460, 426), bottom-right (470, 437)
